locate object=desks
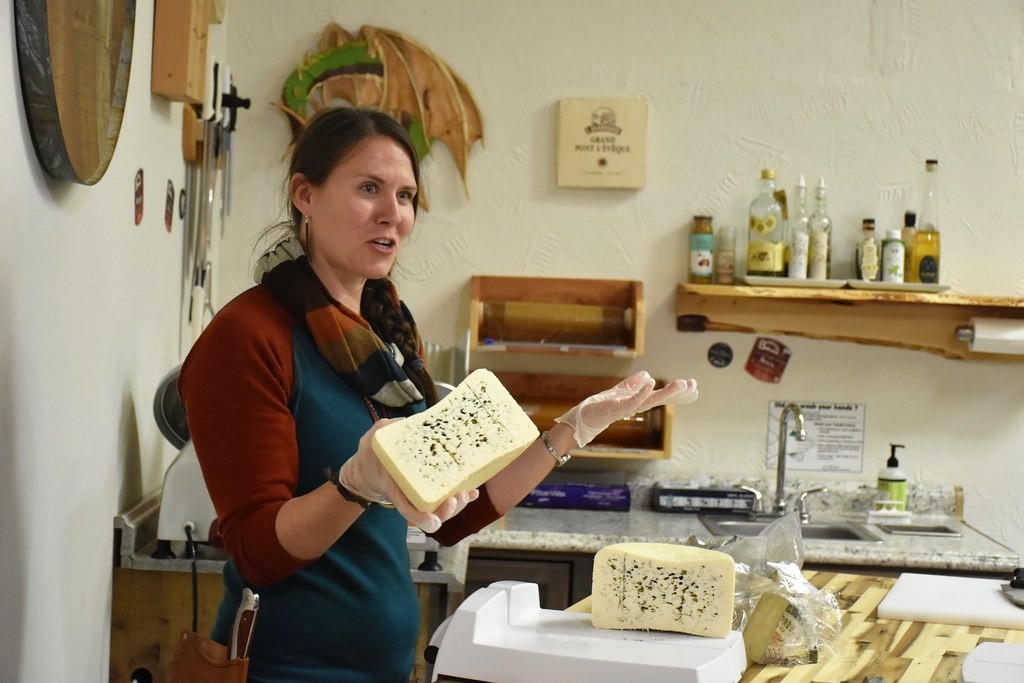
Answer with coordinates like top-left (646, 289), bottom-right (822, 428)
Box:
top-left (560, 569), bottom-right (1024, 683)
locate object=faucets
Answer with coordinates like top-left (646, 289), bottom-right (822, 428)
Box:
top-left (773, 403), bottom-right (807, 511)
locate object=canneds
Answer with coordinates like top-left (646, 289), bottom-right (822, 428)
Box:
top-left (688, 215), bottom-right (714, 283)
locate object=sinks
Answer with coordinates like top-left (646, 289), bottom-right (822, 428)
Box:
top-left (696, 513), bottom-right (884, 544)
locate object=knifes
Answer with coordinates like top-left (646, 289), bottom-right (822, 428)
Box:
top-left (1000, 568), bottom-right (1024, 609)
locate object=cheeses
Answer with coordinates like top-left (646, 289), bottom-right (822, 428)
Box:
top-left (741, 592), bottom-right (811, 666)
top-left (372, 368), bottom-right (541, 513)
top-left (590, 543), bottom-right (737, 642)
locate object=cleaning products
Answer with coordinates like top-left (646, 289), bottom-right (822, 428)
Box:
top-left (876, 442), bottom-right (908, 513)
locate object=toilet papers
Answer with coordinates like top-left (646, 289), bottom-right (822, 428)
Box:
top-left (967, 318), bottom-right (1024, 355)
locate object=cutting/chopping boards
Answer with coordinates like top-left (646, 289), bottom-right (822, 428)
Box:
top-left (877, 573), bottom-right (1024, 631)
top-left (16, 0), bottom-right (137, 185)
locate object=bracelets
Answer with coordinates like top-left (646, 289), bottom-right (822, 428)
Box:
top-left (324, 466), bottom-right (372, 508)
top-left (543, 430), bottom-right (570, 467)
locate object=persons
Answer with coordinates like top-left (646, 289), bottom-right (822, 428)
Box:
top-left (178, 107), bottom-right (698, 683)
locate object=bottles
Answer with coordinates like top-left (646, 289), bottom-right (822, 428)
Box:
top-left (746, 168), bottom-right (784, 278)
top-left (900, 212), bottom-right (918, 282)
top-left (788, 175), bottom-right (809, 280)
top-left (806, 176), bottom-right (833, 280)
top-left (915, 160), bottom-right (941, 284)
top-left (855, 218), bottom-right (882, 282)
top-left (880, 229), bottom-right (906, 284)
top-left (716, 226), bottom-right (736, 285)
top-left (688, 215), bottom-right (714, 285)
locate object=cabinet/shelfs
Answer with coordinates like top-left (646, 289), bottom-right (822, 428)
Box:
top-left (465, 271), bottom-right (673, 460)
